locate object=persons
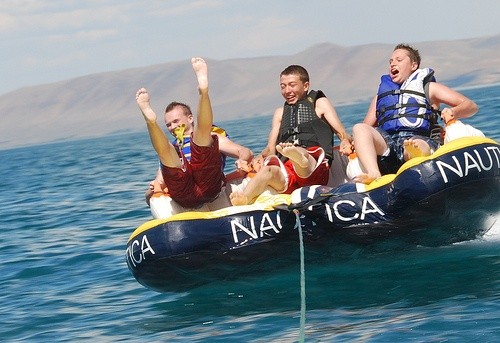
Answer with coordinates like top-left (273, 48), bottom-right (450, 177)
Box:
top-left (136, 57), bottom-right (253, 209)
top-left (342, 45), bottom-right (479, 185)
top-left (229, 65), bottom-right (353, 207)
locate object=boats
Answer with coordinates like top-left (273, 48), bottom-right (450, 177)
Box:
top-left (126, 117), bottom-right (500, 292)
